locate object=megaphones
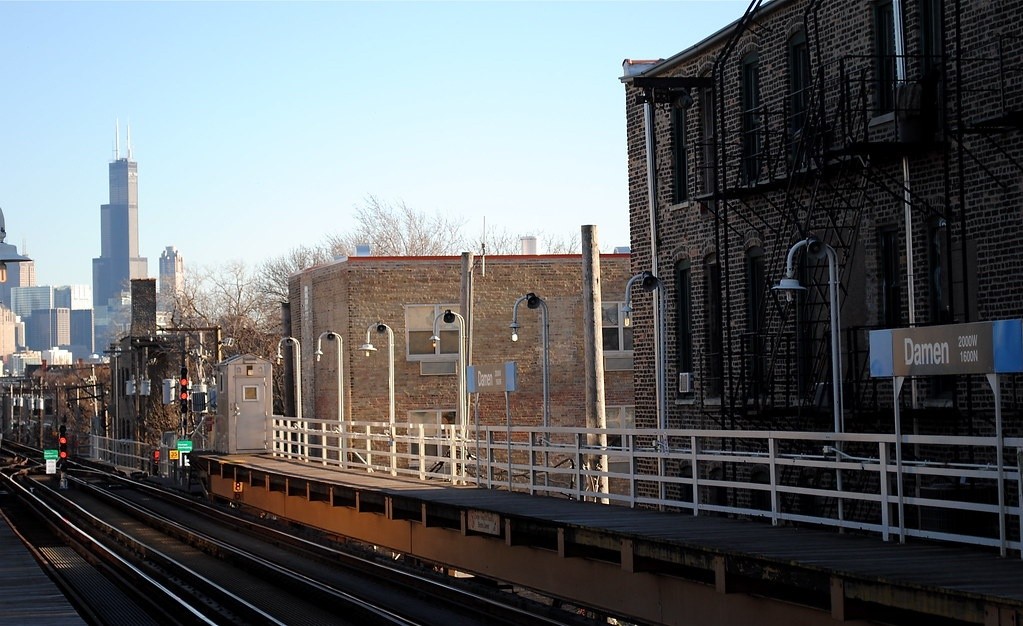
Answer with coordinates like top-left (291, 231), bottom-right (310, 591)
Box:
top-left (377, 319), bottom-right (386, 334)
top-left (642, 272), bottom-right (657, 291)
top-left (526, 293), bottom-right (540, 309)
top-left (285, 337), bottom-right (293, 346)
top-left (327, 330), bottom-right (335, 341)
top-left (806, 238), bottom-right (827, 260)
top-left (444, 309), bottom-right (455, 323)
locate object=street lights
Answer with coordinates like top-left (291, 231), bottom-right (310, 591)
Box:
top-left (619, 270), bottom-right (669, 512)
top-left (358, 319), bottom-right (398, 476)
top-left (771, 238), bottom-right (844, 533)
top-left (276, 337), bottom-right (302, 464)
top-left (315, 331), bottom-right (345, 470)
top-left (429, 309), bottom-right (469, 487)
top-left (508, 293), bottom-right (551, 497)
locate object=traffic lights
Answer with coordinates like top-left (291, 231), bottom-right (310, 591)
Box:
top-left (59, 423), bottom-right (67, 473)
top-left (180, 367), bottom-right (188, 414)
